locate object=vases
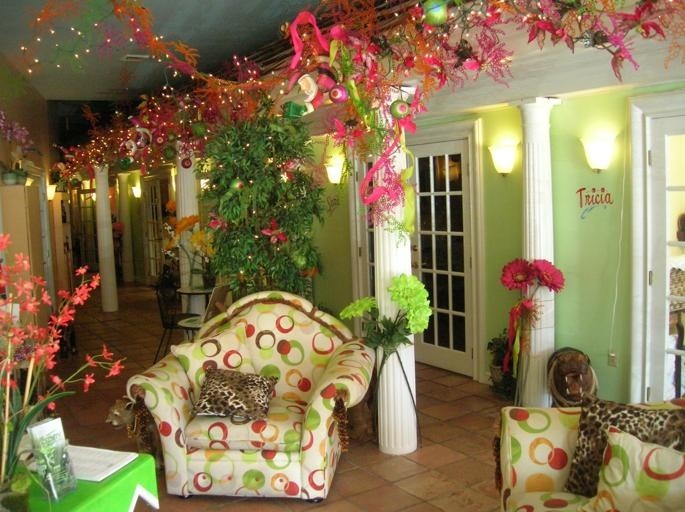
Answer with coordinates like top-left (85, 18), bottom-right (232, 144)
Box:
top-left (16, 169), bottom-right (27, 185)
top-left (1, 171), bottom-right (17, 185)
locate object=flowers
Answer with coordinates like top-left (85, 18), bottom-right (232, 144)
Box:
top-left (163, 200), bottom-right (228, 289)
top-left (500, 252), bottom-right (565, 406)
top-left (0, 111), bottom-right (35, 171)
top-left (341, 274), bottom-right (432, 452)
top-left (0, 234), bottom-right (128, 486)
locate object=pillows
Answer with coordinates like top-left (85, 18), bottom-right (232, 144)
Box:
top-left (190, 367), bottom-right (279, 423)
top-left (563, 392), bottom-right (685, 497)
top-left (170, 318), bottom-right (256, 406)
top-left (579, 426), bottom-right (685, 511)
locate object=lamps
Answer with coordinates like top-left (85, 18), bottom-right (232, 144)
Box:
top-left (580, 132), bottom-right (612, 174)
top-left (132, 186), bottom-right (141, 199)
top-left (323, 162), bottom-right (342, 185)
top-left (46, 184), bottom-right (57, 201)
top-left (91, 192), bottom-right (97, 202)
top-left (489, 141), bottom-right (517, 174)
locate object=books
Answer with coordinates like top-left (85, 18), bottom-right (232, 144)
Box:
top-left (26, 416), bottom-right (67, 462)
top-left (26, 440), bottom-right (139, 482)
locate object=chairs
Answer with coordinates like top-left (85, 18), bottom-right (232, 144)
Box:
top-left (151, 275), bottom-right (201, 365)
top-left (125, 290), bottom-right (377, 503)
top-left (499, 399), bottom-right (685, 512)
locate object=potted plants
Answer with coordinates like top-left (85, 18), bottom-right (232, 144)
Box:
top-left (486, 328), bottom-right (509, 383)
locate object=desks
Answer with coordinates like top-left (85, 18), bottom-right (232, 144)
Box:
top-left (176, 286), bottom-right (216, 312)
top-left (0, 446), bottom-right (160, 512)
